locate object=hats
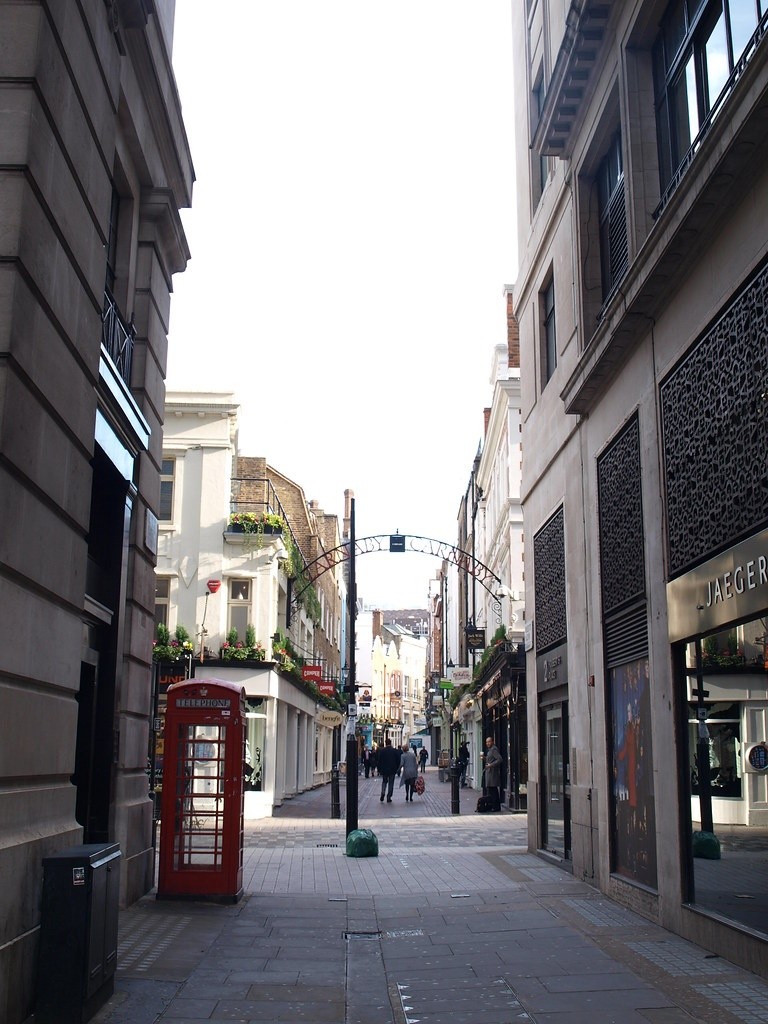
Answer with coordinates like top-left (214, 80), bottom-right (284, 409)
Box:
top-left (461, 741), bottom-right (470, 745)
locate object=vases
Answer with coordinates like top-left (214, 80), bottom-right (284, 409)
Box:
top-left (232, 522), bottom-right (282, 534)
top-left (220, 648), bottom-right (260, 661)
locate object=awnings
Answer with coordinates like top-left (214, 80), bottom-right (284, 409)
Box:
top-left (413, 728), bottom-right (431, 736)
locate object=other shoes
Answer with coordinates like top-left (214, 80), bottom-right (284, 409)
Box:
top-left (387, 800), bottom-right (392, 802)
top-left (461, 783), bottom-right (467, 788)
top-left (410, 799), bottom-right (413, 801)
top-left (380, 793), bottom-right (385, 801)
top-left (406, 796), bottom-right (408, 800)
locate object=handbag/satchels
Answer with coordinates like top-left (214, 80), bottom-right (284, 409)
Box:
top-left (475, 796), bottom-right (492, 812)
top-left (415, 775), bottom-right (425, 795)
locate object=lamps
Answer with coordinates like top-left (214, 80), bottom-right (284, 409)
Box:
top-left (337, 659), bottom-right (352, 685)
top-left (464, 621), bottom-right (488, 635)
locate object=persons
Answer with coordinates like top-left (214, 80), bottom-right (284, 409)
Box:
top-left (419, 746), bottom-right (429, 773)
top-left (362, 739), bottom-right (418, 802)
top-left (459, 742), bottom-right (470, 788)
top-left (485, 737), bottom-right (502, 811)
top-left (413, 745), bottom-right (420, 769)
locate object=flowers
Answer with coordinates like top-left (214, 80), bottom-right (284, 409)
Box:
top-left (232, 511), bottom-right (284, 549)
top-left (701, 645), bottom-right (747, 669)
top-left (152, 622), bottom-right (194, 662)
top-left (222, 623), bottom-right (266, 662)
